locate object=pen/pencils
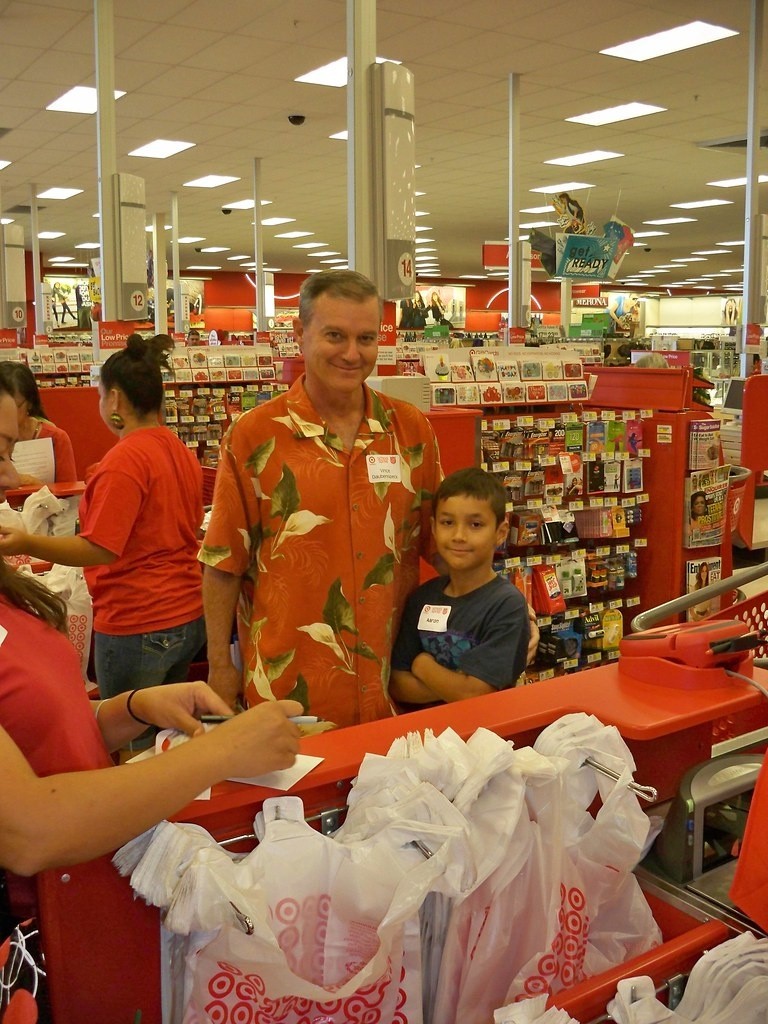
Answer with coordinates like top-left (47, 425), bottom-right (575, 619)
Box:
top-left (201, 715), bottom-right (321, 726)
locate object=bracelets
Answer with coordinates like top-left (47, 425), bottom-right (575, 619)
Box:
top-left (126, 687), bottom-right (152, 729)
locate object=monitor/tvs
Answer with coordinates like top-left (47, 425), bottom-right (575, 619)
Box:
top-left (720, 376), bottom-right (747, 415)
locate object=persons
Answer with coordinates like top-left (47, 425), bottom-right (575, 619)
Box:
top-left (185, 329), bottom-right (201, 347)
top-left (387, 466), bottom-right (532, 715)
top-left (605, 293), bottom-right (640, 332)
top-left (197, 268), bottom-right (445, 731)
top-left (722, 297), bottom-right (740, 326)
top-left (0, 332), bottom-right (206, 768)
top-left (49, 281), bottom-right (83, 328)
top-left (397, 289), bottom-right (455, 330)
top-left (690, 562), bottom-right (712, 621)
top-left (0, 384), bottom-right (304, 931)
top-left (690, 491), bottom-right (708, 520)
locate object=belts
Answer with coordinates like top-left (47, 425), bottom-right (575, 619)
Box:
top-left (696, 610), bottom-right (707, 616)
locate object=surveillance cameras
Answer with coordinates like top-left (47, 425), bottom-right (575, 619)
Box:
top-left (621, 282), bottom-right (625, 284)
top-left (289, 116), bottom-right (305, 126)
top-left (644, 248), bottom-right (651, 252)
top-left (195, 248), bottom-right (202, 252)
top-left (222, 210), bottom-right (231, 215)
